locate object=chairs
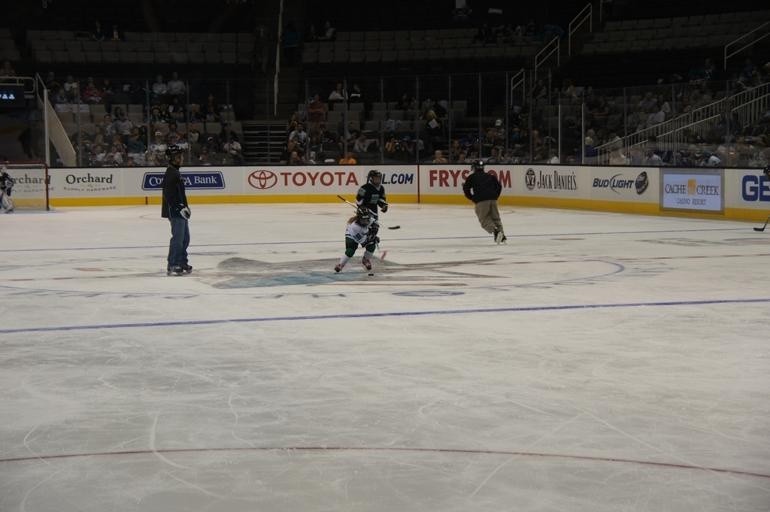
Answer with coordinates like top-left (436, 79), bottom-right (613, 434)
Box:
top-left (54, 101), bottom-right (244, 140)
top-left (324, 99), bottom-right (470, 135)
top-left (0, 9), bottom-right (770, 66)
top-left (542, 94), bottom-right (682, 129)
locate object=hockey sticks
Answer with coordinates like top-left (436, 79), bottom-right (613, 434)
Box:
top-left (375, 240), bottom-right (389, 261)
top-left (337, 195), bottom-right (401, 230)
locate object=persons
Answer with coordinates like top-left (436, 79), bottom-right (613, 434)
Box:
top-left (334, 207), bottom-right (379, 272)
top-left (463, 159), bottom-right (507, 244)
top-left (357, 170), bottom-right (389, 244)
top-left (3, 0), bottom-right (769, 167)
top-left (0, 165), bottom-right (15, 214)
top-left (161, 145), bottom-right (194, 276)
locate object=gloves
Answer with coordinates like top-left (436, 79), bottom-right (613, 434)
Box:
top-left (179, 207), bottom-right (191, 220)
top-left (381, 205), bottom-right (388, 212)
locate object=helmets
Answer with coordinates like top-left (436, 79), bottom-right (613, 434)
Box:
top-left (165, 144), bottom-right (185, 168)
top-left (367, 169), bottom-right (382, 187)
top-left (356, 207), bottom-right (371, 222)
top-left (471, 160), bottom-right (484, 171)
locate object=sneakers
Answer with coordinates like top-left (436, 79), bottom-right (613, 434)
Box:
top-left (167, 264), bottom-right (183, 272)
top-left (501, 236), bottom-right (507, 242)
top-left (494, 229), bottom-right (501, 241)
top-left (362, 256), bottom-right (372, 270)
top-left (183, 263), bottom-right (192, 270)
top-left (335, 263), bottom-right (343, 272)
